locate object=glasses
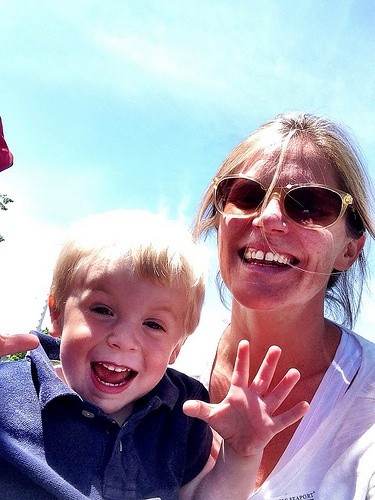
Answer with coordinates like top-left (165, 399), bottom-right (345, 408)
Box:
top-left (213, 175), bottom-right (354, 230)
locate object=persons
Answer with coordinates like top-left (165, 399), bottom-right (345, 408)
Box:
top-left (0, 210), bottom-right (310, 500)
top-left (168, 111), bottom-right (375, 499)
